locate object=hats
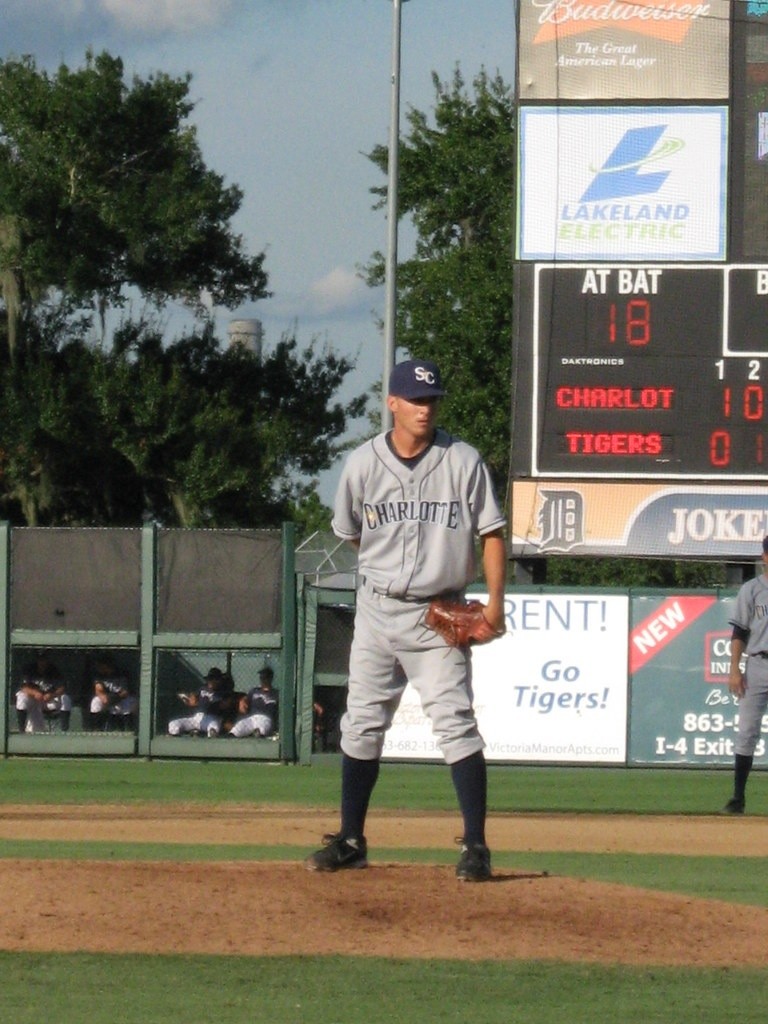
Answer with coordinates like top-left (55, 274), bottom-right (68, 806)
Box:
top-left (258, 668), bottom-right (273, 675)
top-left (203, 668), bottom-right (226, 679)
top-left (390, 361), bottom-right (444, 398)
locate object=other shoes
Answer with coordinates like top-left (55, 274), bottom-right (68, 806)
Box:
top-left (720, 797), bottom-right (745, 815)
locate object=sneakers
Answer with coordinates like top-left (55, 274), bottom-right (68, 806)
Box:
top-left (454, 836), bottom-right (492, 881)
top-left (303, 834), bottom-right (367, 870)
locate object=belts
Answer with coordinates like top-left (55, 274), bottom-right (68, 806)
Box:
top-left (391, 592), bottom-right (466, 604)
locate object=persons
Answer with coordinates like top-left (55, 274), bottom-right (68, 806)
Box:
top-left (16, 649), bottom-right (323, 738)
top-left (723, 537), bottom-right (768, 814)
top-left (305, 362), bottom-right (507, 881)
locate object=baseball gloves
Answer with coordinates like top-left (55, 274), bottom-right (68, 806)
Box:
top-left (416, 600), bottom-right (507, 658)
